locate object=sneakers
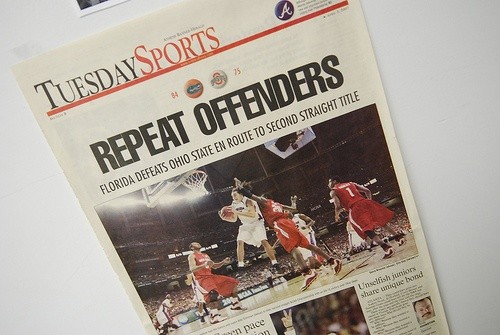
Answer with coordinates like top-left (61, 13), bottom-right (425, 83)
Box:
top-left (200, 316), bottom-right (205, 322)
top-left (333, 259), bottom-right (342, 275)
top-left (300, 271), bottom-right (318, 292)
top-left (398, 237), bottom-right (406, 246)
top-left (321, 268), bottom-right (328, 277)
top-left (274, 263), bottom-right (284, 274)
top-left (382, 246), bottom-right (395, 259)
top-left (231, 302), bottom-right (246, 310)
top-left (168, 327), bottom-right (175, 330)
top-left (346, 257), bottom-right (350, 262)
top-left (235, 265), bottom-right (247, 278)
top-left (366, 248), bottom-right (372, 252)
top-left (210, 314), bottom-right (227, 323)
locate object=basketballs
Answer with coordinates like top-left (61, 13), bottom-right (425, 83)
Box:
top-left (220, 206), bottom-right (234, 219)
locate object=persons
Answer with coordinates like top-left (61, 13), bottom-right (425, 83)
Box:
top-left (414, 297), bottom-right (433, 320)
top-left (118, 174), bottom-right (413, 321)
top-left (158, 294), bottom-right (181, 335)
top-left (281, 309), bottom-right (340, 335)
